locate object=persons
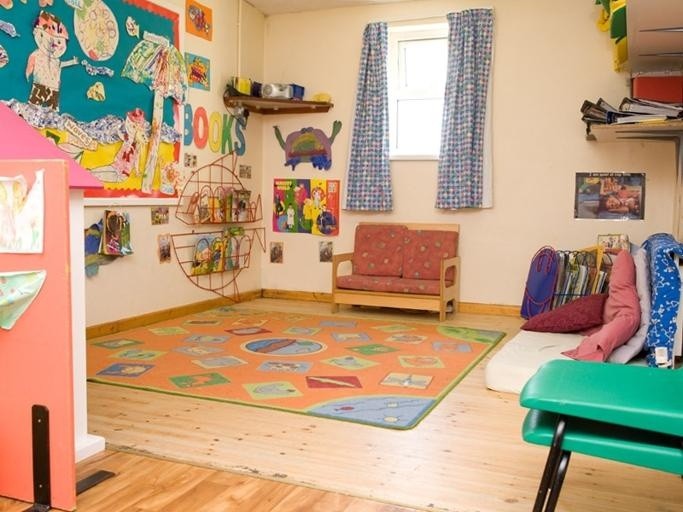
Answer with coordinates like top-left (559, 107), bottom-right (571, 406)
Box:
top-left (25, 10), bottom-right (80, 113)
top-left (275, 192), bottom-right (281, 218)
top-left (89, 107), bottom-right (145, 182)
top-left (313, 187), bottom-right (321, 210)
top-left (286, 203), bottom-right (295, 229)
top-left (297, 183), bottom-right (308, 217)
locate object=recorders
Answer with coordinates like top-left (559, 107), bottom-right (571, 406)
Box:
top-left (261, 82), bottom-right (294, 99)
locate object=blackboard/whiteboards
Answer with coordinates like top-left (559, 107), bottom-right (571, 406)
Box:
top-left (0, 0), bottom-right (188, 206)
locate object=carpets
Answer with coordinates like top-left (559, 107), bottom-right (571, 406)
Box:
top-left (86, 305), bottom-right (508, 430)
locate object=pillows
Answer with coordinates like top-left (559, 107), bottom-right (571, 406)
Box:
top-left (520, 293), bottom-right (607, 334)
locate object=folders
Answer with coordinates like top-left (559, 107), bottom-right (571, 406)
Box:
top-left (579, 97), bottom-right (681, 123)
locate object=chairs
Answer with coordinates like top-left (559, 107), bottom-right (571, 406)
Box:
top-left (520, 359), bottom-right (683, 511)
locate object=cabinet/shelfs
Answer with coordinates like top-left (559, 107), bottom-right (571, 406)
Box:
top-left (590, 1), bottom-right (683, 143)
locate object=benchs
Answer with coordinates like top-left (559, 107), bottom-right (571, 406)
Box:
top-left (330, 221), bottom-right (461, 322)
